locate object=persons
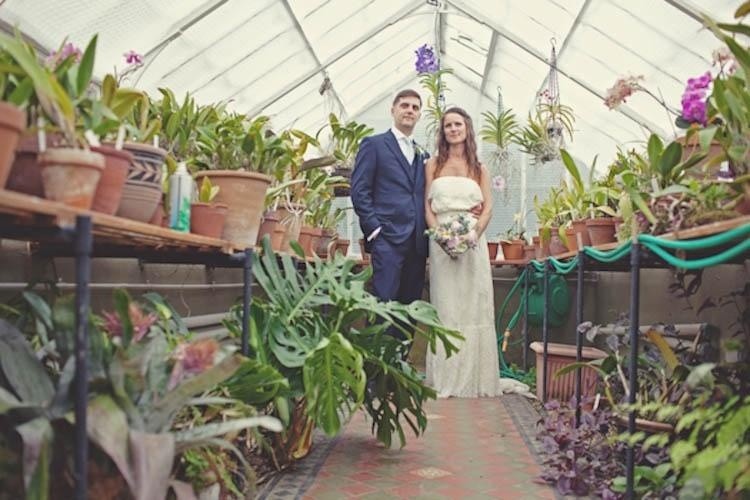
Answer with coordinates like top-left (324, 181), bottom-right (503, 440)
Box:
top-left (424, 106), bottom-right (503, 398)
top-left (351, 89), bottom-right (483, 402)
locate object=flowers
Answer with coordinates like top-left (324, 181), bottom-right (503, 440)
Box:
top-left (682, 23), bottom-right (750, 166)
top-left (29, 37), bottom-right (83, 129)
top-left (506, 200), bottom-right (533, 240)
top-left (601, 47), bottom-right (739, 135)
top-left (77, 50), bottom-right (144, 145)
top-left (44, 81), bottom-right (108, 148)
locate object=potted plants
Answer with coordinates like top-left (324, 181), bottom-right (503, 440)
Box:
top-left (268, 136), bottom-right (307, 251)
top-left (544, 189), bottom-right (567, 255)
top-left (195, 109), bottom-right (272, 249)
top-left (0, 22), bottom-right (47, 190)
top-left (583, 154), bottom-right (617, 248)
top-left (311, 177), bottom-right (350, 258)
top-left (328, 112), bottom-right (375, 196)
top-left (598, 169), bottom-right (651, 242)
top-left (257, 179), bottom-right (305, 246)
top-left (102, 94), bottom-right (168, 223)
top-left (149, 141), bottom-right (175, 226)
top-left (559, 147), bottom-right (592, 249)
top-left (557, 181), bottom-right (578, 251)
top-left (298, 169), bottom-right (326, 255)
top-left (532, 194), bottom-right (550, 262)
top-left (316, 208), bottom-right (346, 258)
top-left (191, 176), bottom-right (230, 239)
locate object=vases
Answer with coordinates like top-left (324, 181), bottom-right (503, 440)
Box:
top-left (677, 136), bottom-right (728, 181)
top-left (35, 150), bottom-right (107, 211)
top-left (726, 167), bottom-right (749, 216)
top-left (533, 342), bottom-right (609, 406)
top-left (88, 146), bottom-right (133, 215)
top-left (7, 126), bottom-right (63, 197)
top-left (488, 242), bottom-right (498, 260)
top-left (336, 239), bottom-right (351, 256)
top-left (358, 238), bottom-right (369, 260)
top-left (500, 240), bottom-right (526, 260)
